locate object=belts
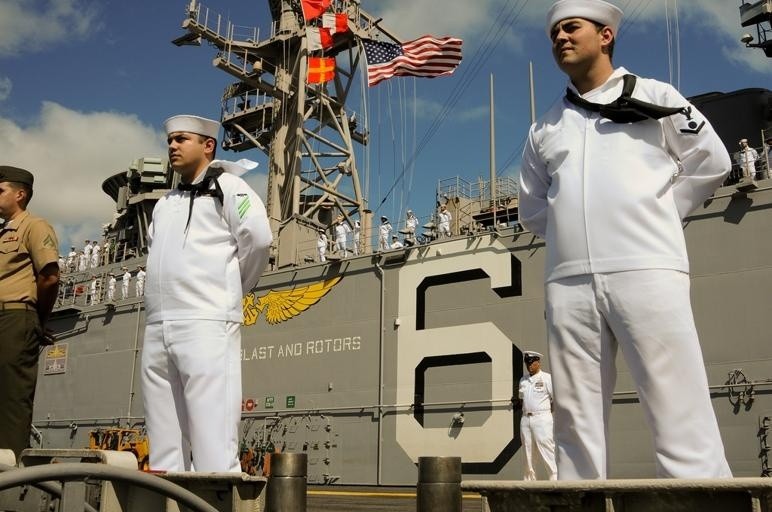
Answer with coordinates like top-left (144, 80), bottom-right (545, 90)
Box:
top-left (0, 303), bottom-right (37, 312)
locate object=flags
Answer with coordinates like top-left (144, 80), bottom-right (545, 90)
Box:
top-left (359, 35), bottom-right (465, 89)
top-left (305, 25), bottom-right (334, 53)
top-left (320, 11), bottom-right (351, 37)
top-left (300, 0), bottom-right (332, 23)
top-left (306, 55), bottom-right (336, 85)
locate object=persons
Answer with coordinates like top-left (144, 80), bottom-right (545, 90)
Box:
top-left (134, 264), bottom-right (145, 296)
top-left (515, 0), bottom-right (738, 479)
top-left (316, 227), bottom-right (328, 263)
top-left (89, 274), bottom-right (98, 306)
top-left (78, 249), bottom-right (86, 272)
top-left (391, 234), bottom-right (404, 248)
top-left (735, 138), bottom-right (760, 180)
top-left (0, 164), bottom-right (61, 468)
top-left (376, 214), bottom-right (393, 252)
top-left (335, 215), bottom-right (351, 258)
top-left (58, 254), bottom-right (64, 273)
top-left (138, 113), bottom-right (275, 474)
top-left (437, 205), bottom-right (453, 240)
top-left (82, 239), bottom-right (94, 269)
top-left (107, 272), bottom-right (116, 302)
top-left (65, 246), bottom-right (76, 273)
top-left (352, 220), bottom-right (361, 256)
top-left (120, 266), bottom-right (132, 300)
top-left (406, 209), bottom-right (419, 246)
top-left (516, 349), bottom-right (560, 481)
top-left (90, 241), bottom-right (101, 270)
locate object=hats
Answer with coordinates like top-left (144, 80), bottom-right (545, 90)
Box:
top-left (522, 351), bottom-right (543, 361)
top-left (0, 166), bottom-right (34, 186)
top-left (545, 0), bottom-right (624, 44)
top-left (318, 204), bottom-right (446, 239)
top-left (58, 239), bottom-right (97, 259)
top-left (92, 265), bottom-right (143, 278)
top-left (163, 114), bottom-right (221, 142)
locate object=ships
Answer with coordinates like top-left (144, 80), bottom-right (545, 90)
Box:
top-left (0, 1), bottom-right (772, 511)
